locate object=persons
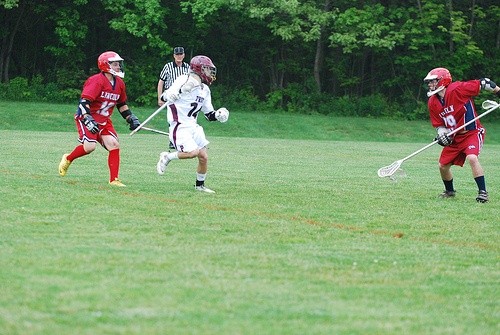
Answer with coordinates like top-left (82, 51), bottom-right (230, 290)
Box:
top-left (158, 47), bottom-right (190, 149)
top-left (157, 56), bottom-right (230, 193)
top-left (59, 51), bottom-right (141, 186)
top-left (424, 68), bottom-right (500, 203)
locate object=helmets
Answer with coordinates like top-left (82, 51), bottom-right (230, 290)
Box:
top-left (190, 55), bottom-right (217, 87)
top-left (97, 51), bottom-right (125, 79)
top-left (424, 67), bottom-right (452, 98)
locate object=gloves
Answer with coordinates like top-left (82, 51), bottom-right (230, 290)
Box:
top-left (126, 114), bottom-right (141, 132)
top-left (81, 115), bottom-right (100, 134)
top-left (434, 127), bottom-right (453, 146)
top-left (163, 90), bottom-right (182, 104)
top-left (214, 107), bottom-right (230, 123)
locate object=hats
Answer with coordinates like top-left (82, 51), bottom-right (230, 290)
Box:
top-left (174, 47), bottom-right (184, 54)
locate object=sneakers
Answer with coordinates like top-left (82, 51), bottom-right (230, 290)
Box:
top-left (59, 153), bottom-right (72, 177)
top-left (475, 190), bottom-right (489, 203)
top-left (156, 151), bottom-right (171, 175)
top-left (438, 190), bottom-right (457, 198)
top-left (109, 177), bottom-right (126, 187)
top-left (194, 184), bottom-right (216, 194)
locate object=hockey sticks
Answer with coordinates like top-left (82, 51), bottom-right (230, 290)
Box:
top-left (129, 79), bottom-right (204, 136)
top-left (378, 99), bottom-right (500, 182)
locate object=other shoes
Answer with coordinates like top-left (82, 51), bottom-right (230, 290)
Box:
top-left (169, 141), bottom-right (175, 149)
